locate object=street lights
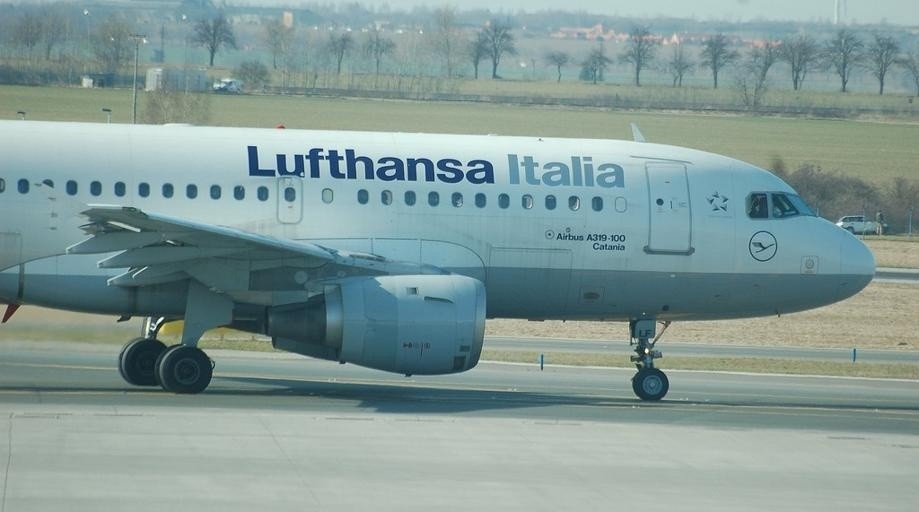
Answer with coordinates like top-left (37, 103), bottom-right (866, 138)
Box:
top-left (82, 8), bottom-right (91, 60)
top-left (181, 13), bottom-right (188, 63)
top-left (17, 111), bottom-right (26, 120)
top-left (126, 35), bottom-right (149, 124)
top-left (102, 107), bottom-right (111, 123)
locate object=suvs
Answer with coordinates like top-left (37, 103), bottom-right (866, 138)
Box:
top-left (211, 78), bottom-right (242, 95)
top-left (834, 215), bottom-right (889, 235)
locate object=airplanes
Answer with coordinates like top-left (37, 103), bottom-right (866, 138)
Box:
top-left (1, 115), bottom-right (875, 405)
